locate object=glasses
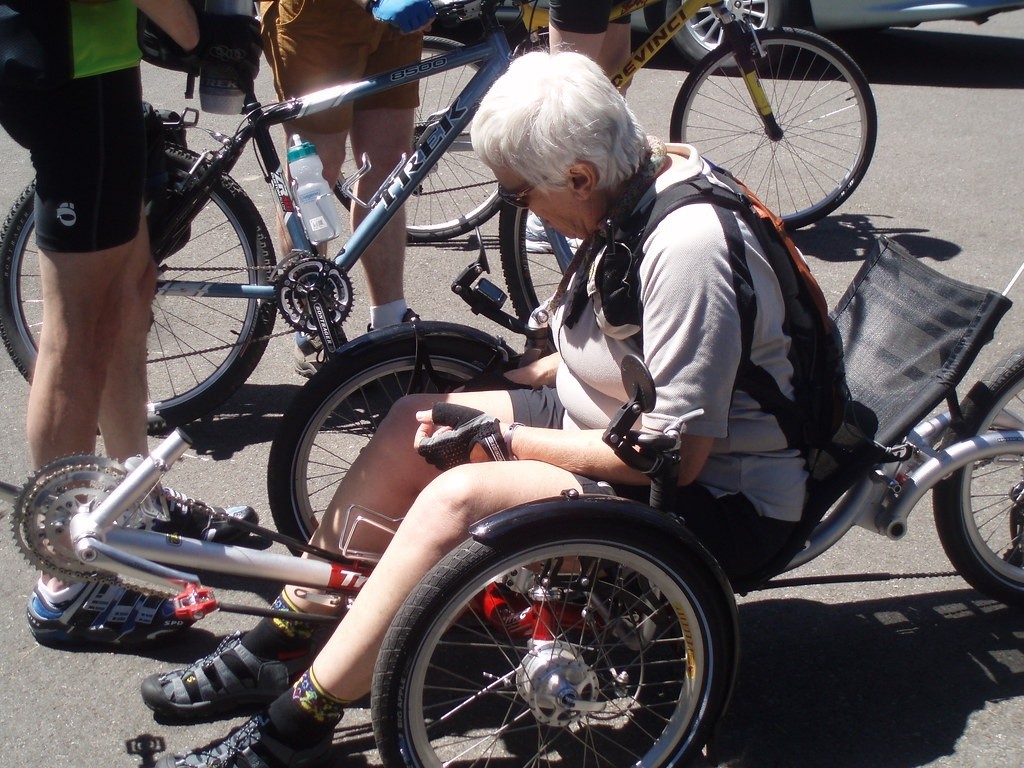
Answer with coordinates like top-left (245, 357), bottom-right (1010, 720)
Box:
top-left (499, 172), bottom-right (552, 208)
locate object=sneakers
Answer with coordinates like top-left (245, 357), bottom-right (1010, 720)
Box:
top-left (27, 573), bottom-right (199, 644)
top-left (136, 486), bottom-right (259, 546)
top-left (294, 323), bottom-right (348, 376)
top-left (151, 707), bottom-right (345, 768)
top-left (142, 632), bottom-right (293, 716)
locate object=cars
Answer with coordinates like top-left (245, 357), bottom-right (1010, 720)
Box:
top-left (415, 0), bottom-right (1023, 78)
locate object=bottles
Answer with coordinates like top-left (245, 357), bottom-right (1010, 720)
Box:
top-left (288, 134), bottom-right (340, 243)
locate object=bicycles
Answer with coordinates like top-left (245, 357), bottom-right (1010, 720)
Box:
top-left (320, 0), bottom-right (878, 239)
top-left (0, 0), bottom-right (718, 436)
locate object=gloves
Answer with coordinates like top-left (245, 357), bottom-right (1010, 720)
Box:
top-left (183, 14), bottom-right (265, 76)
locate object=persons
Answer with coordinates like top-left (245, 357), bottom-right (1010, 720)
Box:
top-left (140, 51), bottom-right (847, 768)
top-left (0, 1), bottom-right (263, 650)
top-left (255, 0), bottom-right (437, 376)
top-left (523, 0), bottom-right (632, 254)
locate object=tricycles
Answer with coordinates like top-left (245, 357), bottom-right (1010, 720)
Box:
top-left (4, 205), bottom-right (1024, 768)
